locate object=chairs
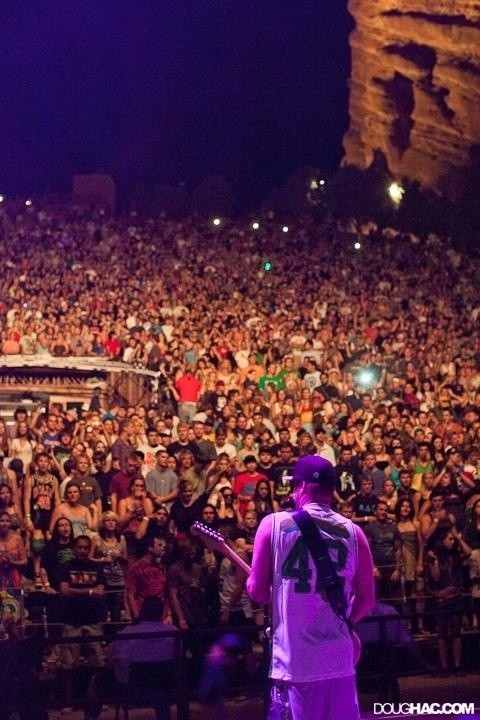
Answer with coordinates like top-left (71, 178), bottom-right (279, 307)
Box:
top-left (79, 638), bottom-right (407, 720)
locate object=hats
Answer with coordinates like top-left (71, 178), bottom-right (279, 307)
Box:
top-left (283, 456), bottom-right (336, 485)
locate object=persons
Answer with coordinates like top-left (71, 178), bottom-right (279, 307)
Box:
top-left (0, 198), bottom-right (480, 720)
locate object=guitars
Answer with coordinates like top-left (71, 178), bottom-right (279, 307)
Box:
top-left (191, 522), bottom-right (271, 599)
